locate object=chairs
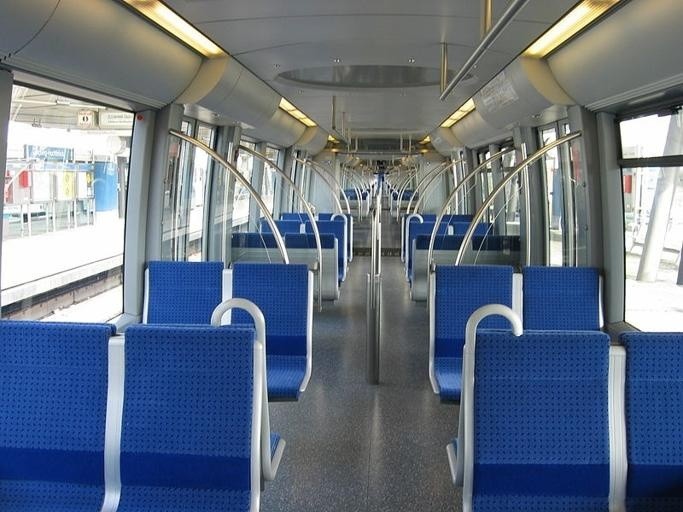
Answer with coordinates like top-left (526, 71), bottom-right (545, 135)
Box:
top-left (133, 260), bottom-right (315, 403)
top-left (3, 291), bottom-right (286, 510)
top-left (340, 180), bottom-right (419, 214)
top-left (428, 266), bottom-right (605, 406)
top-left (227, 211), bottom-right (352, 301)
top-left (402, 213), bottom-right (522, 302)
top-left (441, 303), bottom-right (683, 512)
